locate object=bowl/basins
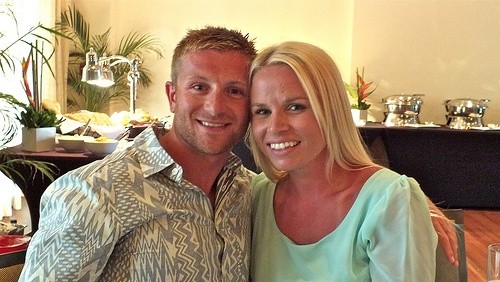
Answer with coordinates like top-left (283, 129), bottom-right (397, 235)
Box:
top-left (85, 139), bottom-right (118, 156)
top-left (0, 235), bottom-right (30, 253)
top-left (58, 136), bottom-right (93, 152)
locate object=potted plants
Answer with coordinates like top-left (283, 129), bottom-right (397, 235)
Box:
top-left (343, 102), bottom-right (371, 124)
top-left (17, 105), bottom-right (67, 153)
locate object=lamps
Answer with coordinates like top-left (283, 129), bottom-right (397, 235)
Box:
top-left (81, 47), bottom-right (140, 114)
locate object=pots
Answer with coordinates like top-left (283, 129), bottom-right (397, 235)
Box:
top-left (381, 93), bottom-right (423, 115)
top-left (444, 98), bottom-right (490, 116)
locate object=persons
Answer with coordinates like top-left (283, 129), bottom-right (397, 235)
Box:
top-left (17, 26), bottom-right (459, 282)
top-left (243, 41), bottom-right (437, 282)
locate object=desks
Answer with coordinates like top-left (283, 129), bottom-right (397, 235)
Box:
top-left (0, 143), bottom-right (106, 237)
top-left (356, 122), bottom-right (500, 211)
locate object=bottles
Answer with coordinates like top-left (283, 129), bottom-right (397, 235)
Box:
top-left (10, 220), bottom-right (19, 230)
